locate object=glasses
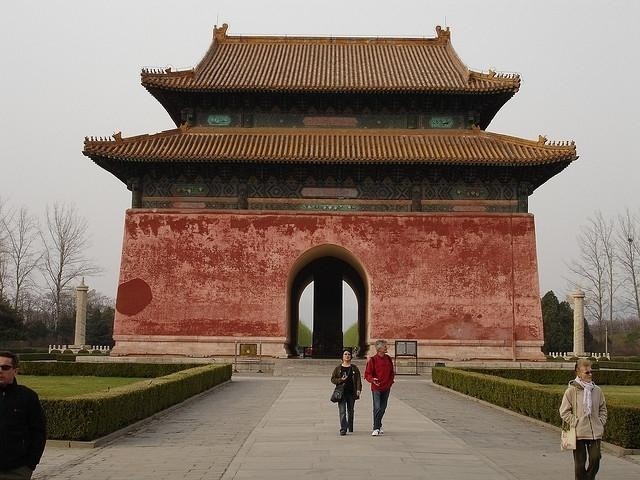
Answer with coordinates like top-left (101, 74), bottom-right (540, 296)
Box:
top-left (0, 365), bottom-right (16, 370)
top-left (581, 371), bottom-right (593, 375)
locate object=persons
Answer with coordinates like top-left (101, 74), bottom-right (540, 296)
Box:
top-left (364, 339), bottom-right (394, 436)
top-left (558, 359), bottom-right (608, 480)
top-left (330, 349), bottom-right (362, 436)
top-left (0, 350), bottom-right (47, 480)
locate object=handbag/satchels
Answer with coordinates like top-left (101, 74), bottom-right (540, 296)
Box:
top-left (560, 414), bottom-right (578, 452)
top-left (330, 385), bottom-right (345, 402)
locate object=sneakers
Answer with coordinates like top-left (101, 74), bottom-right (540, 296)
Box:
top-left (340, 427), bottom-right (353, 436)
top-left (371, 428), bottom-right (384, 436)
